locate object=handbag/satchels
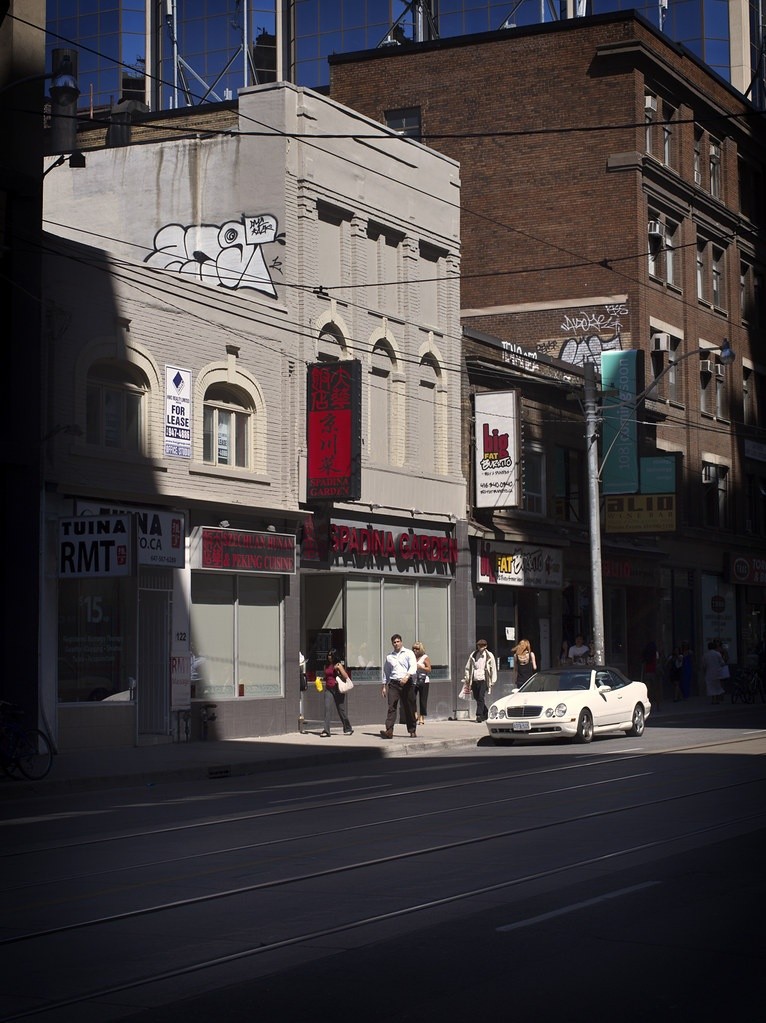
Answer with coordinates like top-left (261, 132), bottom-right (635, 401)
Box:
top-left (336, 675), bottom-right (354, 694)
top-left (717, 665), bottom-right (730, 679)
top-left (512, 654), bottom-right (519, 684)
top-left (458, 679), bottom-right (474, 701)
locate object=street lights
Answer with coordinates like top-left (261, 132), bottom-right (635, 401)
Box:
top-left (586, 336), bottom-right (737, 663)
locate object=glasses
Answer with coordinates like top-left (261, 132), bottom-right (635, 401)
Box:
top-left (588, 642), bottom-right (594, 644)
top-left (413, 647), bottom-right (421, 650)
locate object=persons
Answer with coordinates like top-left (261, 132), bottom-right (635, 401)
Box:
top-left (381, 634), bottom-right (418, 739)
top-left (664, 638), bottom-right (729, 705)
top-left (568, 635), bottom-right (594, 666)
top-left (411, 642), bottom-right (432, 725)
top-left (358, 643), bottom-right (374, 670)
top-left (640, 641), bottom-right (663, 712)
top-left (319, 649), bottom-right (355, 737)
top-left (511, 639), bottom-right (537, 689)
top-left (464, 639), bottom-right (497, 723)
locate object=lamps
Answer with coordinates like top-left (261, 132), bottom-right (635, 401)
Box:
top-left (412, 508), bottom-right (424, 515)
top-left (218, 520), bottom-right (230, 528)
top-left (370, 503), bottom-right (383, 508)
top-left (267, 526), bottom-right (276, 533)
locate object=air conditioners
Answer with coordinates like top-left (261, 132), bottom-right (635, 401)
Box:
top-left (651, 333), bottom-right (670, 352)
top-left (715, 364), bottom-right (726, 377)
top-left (709, 146), bottom-right (720, 161)
top-left (700, 360), bottom-right (714, 373)
top-left (648, 221), bottom-right (665, 237)
top-left (644, 96), bottom-right (657, 113)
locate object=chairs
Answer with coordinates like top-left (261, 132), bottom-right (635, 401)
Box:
top-left (570, 676), bottom-right (588, 687)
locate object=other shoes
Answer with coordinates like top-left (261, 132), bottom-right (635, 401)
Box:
top-left (415, 715), bottom-right (418, 721)
top-left (419, 718), bottom-right (424, 725)
top-left (410, 732), bottom-right (416, 738)
top-left (476, 713), bottom-right (488, 722)
top-left (344, 730), bottom-right (354, 735)
top-left (320, 731), bottom-right (330, 737)
top-left (380, 731), bottom-right (393, 739)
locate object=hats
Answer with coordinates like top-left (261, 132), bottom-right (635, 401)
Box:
top-left (476, 639), bottom-right (487, 645)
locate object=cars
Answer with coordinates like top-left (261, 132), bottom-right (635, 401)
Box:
top-left (58, 651), bottom-right (119, 703)
top-left (484, 663), bottom-right (653, 745)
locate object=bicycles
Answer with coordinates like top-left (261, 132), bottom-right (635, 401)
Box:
top-left (730, 667), bottom-right (760, 705)
top-left (0, 700), bottom-right (54, 781)
